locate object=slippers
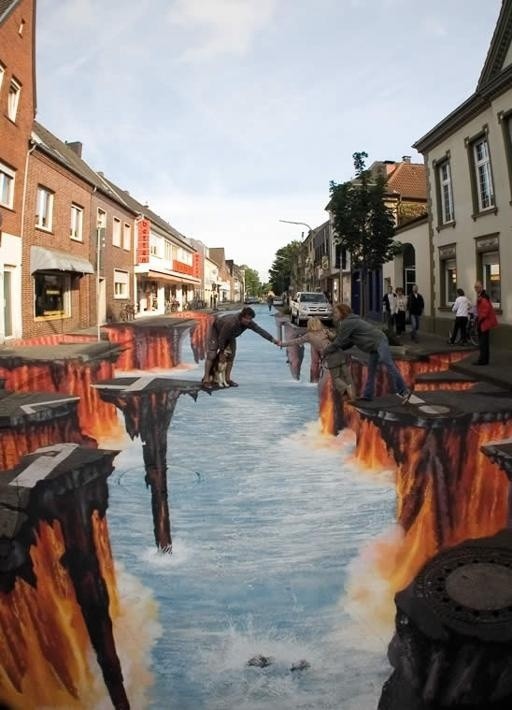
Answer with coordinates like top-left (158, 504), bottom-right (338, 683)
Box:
top-left (202, 377), bottom-right (213, 389)
top-left (225, 380), bottom-right (238, 387)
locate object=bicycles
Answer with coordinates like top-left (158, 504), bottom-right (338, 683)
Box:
top-left (446, 308), bottom-right (479, 348)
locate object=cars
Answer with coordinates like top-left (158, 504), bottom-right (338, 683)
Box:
top-left (273, 297), bottom-right (284, 306)
top-left (245, 296), bottom-right (260, 304)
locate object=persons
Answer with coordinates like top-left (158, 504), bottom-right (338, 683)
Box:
top-left (280, 317), bottom-right (357, 401)
top-left (447, 287), bottom-right (472, 346)
top-left (201, 307), bottom-right (281, 389)
top-left (267, 294), bottom-right (274, 312)
top-left (474, 281), bottom-right (498, 365)
top-left (383, 285), bottom-right (424, 340)
top-left (320, 303), bottom-right (412, 406)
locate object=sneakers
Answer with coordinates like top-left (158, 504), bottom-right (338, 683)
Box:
top-left (356, 396), bottom-right (373, 402)
top-left (401, 386), bottom-right (414, 405)
top-left (396, 332), bottom-right (491, 367)
top-left (347, 384), bottom-right (356, 401)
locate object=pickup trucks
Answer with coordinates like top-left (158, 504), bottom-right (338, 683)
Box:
top-left (289, 291), bottom-right (334, 327)
